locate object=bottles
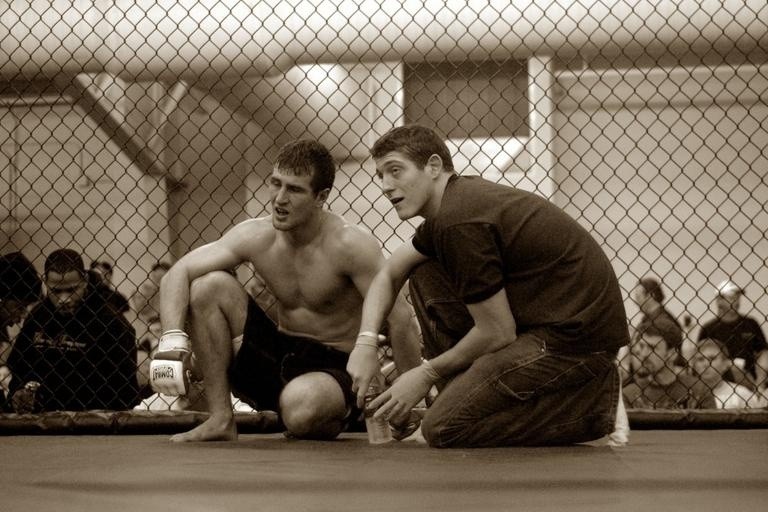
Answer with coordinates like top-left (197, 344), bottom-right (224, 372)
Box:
top-left (22, 381), bottom-right (41, 413)
top-left (363, 374), bottom-right (392, 444)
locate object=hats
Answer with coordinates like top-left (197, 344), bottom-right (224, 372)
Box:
top-left (713, 281), bottom-right (745, 296)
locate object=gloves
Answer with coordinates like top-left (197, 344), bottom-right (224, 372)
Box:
top-left (149, 330), bottom-right (204, 399)
top-left (347, 332), bottom-right (385, 407)
top-left (367, 360), bottom-right (438, 423)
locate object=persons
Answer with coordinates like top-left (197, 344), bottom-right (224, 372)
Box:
top-left (130, 289), bottom-right (156, 324)
top-left (149, 266), bottom-right (169, 323)
top-left (146, 318), bottom-right (162, 363)
top-left (694, 340), bottom-right (756, 409)
top-left (91, 261), bottom-right (110, 289)
top-left (345, 125), bottom-right (630, 448)
top-left (149, 141), bottom-right (422, 442)
top-left (620, 321), bottom-right (716, 409)
top-left (628, 279), bottom-right (682, 365)
top-left (0, 252), bottom-right (42, 332)
top-left (0, 250), bottom-right (138, 415)
top-left (699, 282), bottom-right (767, 399)
